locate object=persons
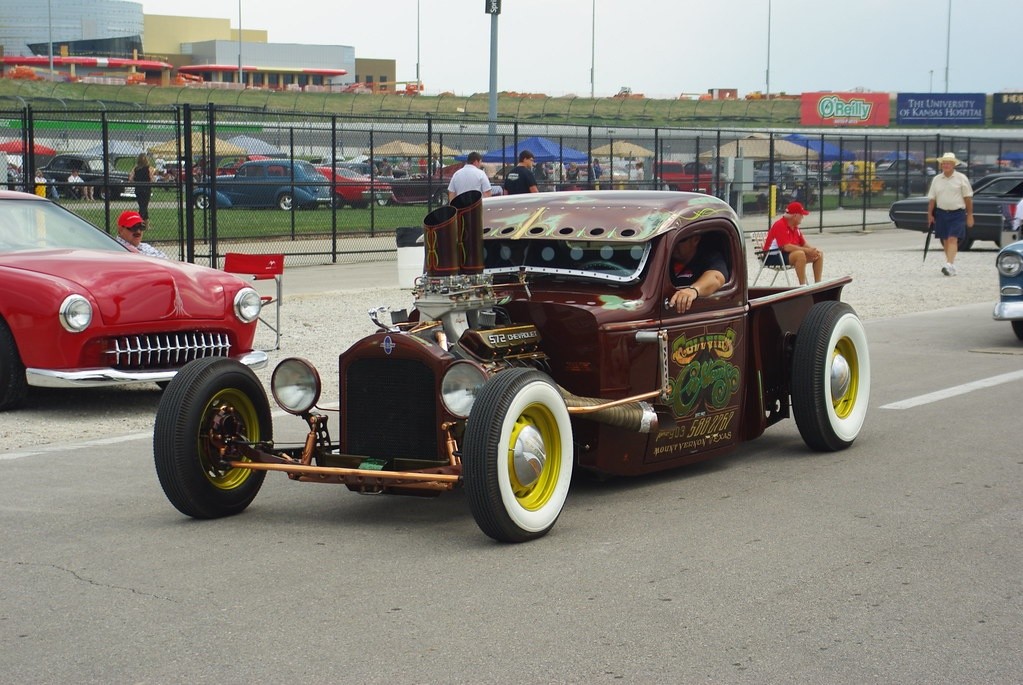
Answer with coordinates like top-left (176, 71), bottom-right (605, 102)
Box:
top-left (531, 158), bottom-right (670, 190)
top-left (502, 150), bottom-right (538, 195)
top-left (667, 232), bottom-right (728, 314)
top-left (116, 211), bottom-right (167, 260)
top-left (927, 151), bottom-right (973, 275)
top-left (154, 155), bottom-right (166, 172)
top-left (68, 170), bottom-right (94, 201)
top-left (760, 202), bottom-right (823, 284)
top-left (129, 153), bottom-right (152, 229)
top-left (845, 161), bottom-right (855, 184)
top-left (366, 155), bottom-right (440, 177)
top-left (447, 152), bottom-right (491, 204)
top-left (35, 170), bottom-right (58, 201)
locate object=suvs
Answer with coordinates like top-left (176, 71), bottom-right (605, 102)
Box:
top-left (650, 161), bottom-right (712, 193)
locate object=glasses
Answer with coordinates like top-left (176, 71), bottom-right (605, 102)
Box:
top-left (123, 224), bottom-right (147, 231)
top-left (679, 236), bottom-right (691, 243)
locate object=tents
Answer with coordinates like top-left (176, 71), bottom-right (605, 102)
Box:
top-left (0, 131), bottom-right (1023, 174)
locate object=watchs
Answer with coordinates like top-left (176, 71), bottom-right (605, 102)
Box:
top-left (688, 286), bottom-right (698, 298)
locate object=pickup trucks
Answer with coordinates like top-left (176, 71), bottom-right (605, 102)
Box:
top-left (153, 189), bottom-right (872, 544)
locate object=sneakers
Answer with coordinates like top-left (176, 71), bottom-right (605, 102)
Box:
top-left (942, 264), bottom-right (957, 276)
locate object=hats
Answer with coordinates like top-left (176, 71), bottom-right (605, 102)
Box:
top-left (937, 153), bottom-right (960, 166)
top-left (119, 212), bottom-right (142, 228)
top-left (786, 202), bottom-right (809, 215)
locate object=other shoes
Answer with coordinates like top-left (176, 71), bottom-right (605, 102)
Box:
top-left (145, 217), bottom-right (150, 230)
top-left (85, 198), bottom-right (96, 203)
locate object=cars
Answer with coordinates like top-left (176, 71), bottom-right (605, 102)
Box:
top-left (156, 159), bottom-right (199, 181)
top-left (193, 160), bottom-right (333, 211)
top-left (992, 240), bottom-right (1023, 340)
top-left (316, 167), bottom-right (393, 209)
top-left (376, 175), bottom-right (453, 206)
top-left (0, 189), bottom-right (262, 413)
top-left (755, 163), bottom-right (826, 187)
top-left (874, 158), bottom-right (1013, 191)
top-left (37, 153), bottom-right (132, 200)
top-left (218, 163), bottom-right (244, 175)
top-left (889, 172), bottom-right (1023, 251)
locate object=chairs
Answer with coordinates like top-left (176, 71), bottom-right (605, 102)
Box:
top-left (63, 180), bottom-right (80, 202)
top-left (750, 232), bottom-right (809, 287)
top-left (223, 251), bottom-right (286, 351)
